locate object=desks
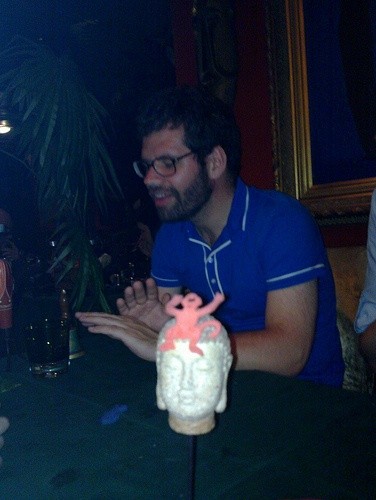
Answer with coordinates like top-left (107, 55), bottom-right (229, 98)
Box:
top-left (0, 336), bottom-right (376, 500)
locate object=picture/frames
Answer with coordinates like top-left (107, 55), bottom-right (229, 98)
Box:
top-left (267, 0), bottom-right (376, 219)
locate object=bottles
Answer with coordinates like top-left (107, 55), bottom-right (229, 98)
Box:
top-left (109, 272), bottom-right (126, 315)
top-left (51, 289), bottom-right (79, 359)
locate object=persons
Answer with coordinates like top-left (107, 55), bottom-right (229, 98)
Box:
top-left (75, 86), bottom-right (345, 391)
top-left (154, 292), bottom-right (234, 436)
top-left (353, 182), bottom-right (375, 391)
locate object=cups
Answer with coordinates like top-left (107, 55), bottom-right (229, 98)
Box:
top-left (25, 318), bottom-right (71, 378)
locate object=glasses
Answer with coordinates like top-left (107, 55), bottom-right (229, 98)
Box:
top-left (132, 151), bottom-right (194, 178)
